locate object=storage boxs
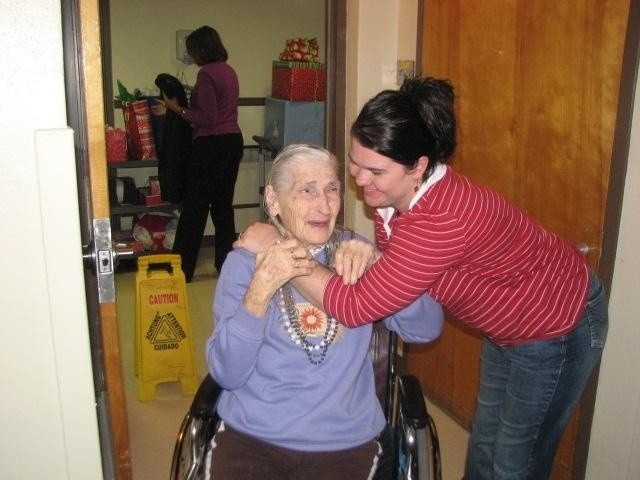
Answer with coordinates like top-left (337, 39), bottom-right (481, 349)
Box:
top-left (263, 95), bottom-right (326, 152)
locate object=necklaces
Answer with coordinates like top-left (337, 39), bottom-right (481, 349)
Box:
top-left (272, 232), bottom-right (343, 366)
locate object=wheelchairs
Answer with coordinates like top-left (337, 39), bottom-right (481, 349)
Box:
top-left (169, 319), bottom-right (442, 480)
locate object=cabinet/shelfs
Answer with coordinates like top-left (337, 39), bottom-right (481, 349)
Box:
top-left (107, 143), bottom-right (269, 231)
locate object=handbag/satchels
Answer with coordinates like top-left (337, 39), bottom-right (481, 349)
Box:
top-left (121, 99), bottom-right (159, 161)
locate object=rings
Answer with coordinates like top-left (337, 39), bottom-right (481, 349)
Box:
top-left (293, 258), bottom-right (303, 267)
top-left (290, 251), bottom-right (298, 259)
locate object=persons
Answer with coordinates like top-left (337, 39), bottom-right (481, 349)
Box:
top-left (232, 76), bottom-right (609, 477)
top-left (205, 141), bottom-right (444, 477)
top-left (148, 25), bottom-right (240, 285)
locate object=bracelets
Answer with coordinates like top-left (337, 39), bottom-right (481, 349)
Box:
top-left (178, 105), bottom-right (186, 117)
